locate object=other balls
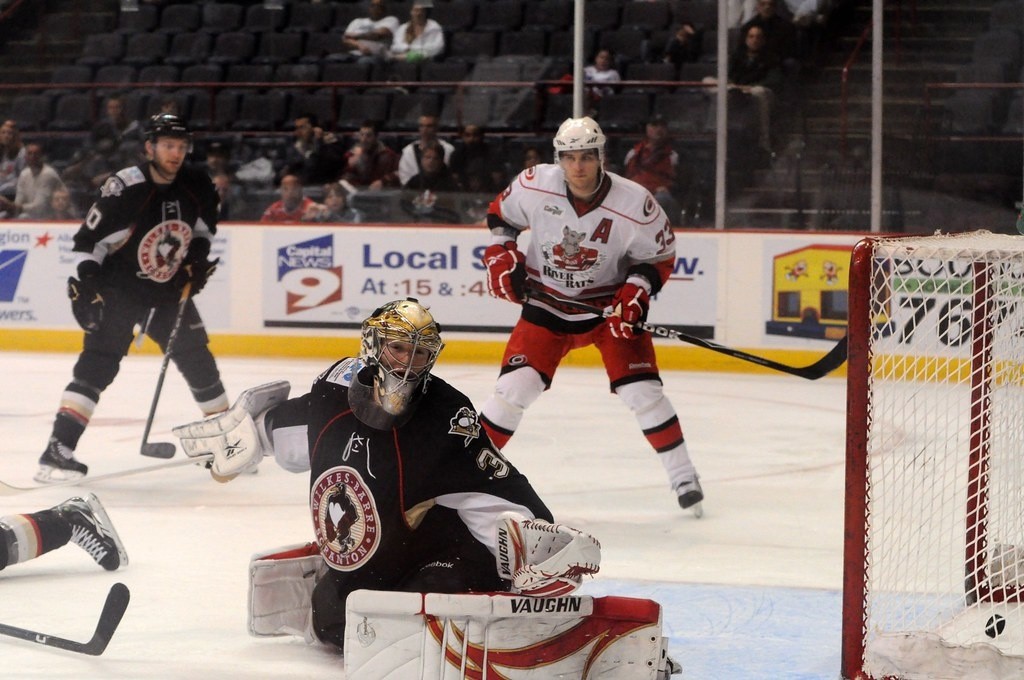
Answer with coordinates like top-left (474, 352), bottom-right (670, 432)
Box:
top-left (985, 614), bottom-right (1006, 638)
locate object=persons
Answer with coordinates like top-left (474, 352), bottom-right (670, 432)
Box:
top-left (170, 298), bottom-right (685, 680)
top-left (0, 493), bottom-right (130, 578)
top-left (31, 110), bottom-right (260, 479)
top-left (461, 116), bottom-right (703, 519)
top-left (0, 0), bottom-right (850, 221)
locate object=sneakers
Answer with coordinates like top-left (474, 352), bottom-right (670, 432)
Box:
top-left (52, 492), bottom-right (129, 571)
top-left (35, 434), bottom-right (88, 485)
top-left (672, 474), bottom-right (704, 518)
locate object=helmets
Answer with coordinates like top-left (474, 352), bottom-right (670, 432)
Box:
top-left (553, 117), bottom-right (606, 168)
top-left (360, 298), bottom-right (445, 416)
top-left (142, 113), bottom-right (193, 145)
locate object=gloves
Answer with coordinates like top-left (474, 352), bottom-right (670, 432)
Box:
top-left (605, 283), bottom-right (649, 340)
top-left (66, 260), bottom-right (107, 335)
top-left (479, 241), bottom-right (529, 305)
top-left (176, 236), bottom-right (220, 299)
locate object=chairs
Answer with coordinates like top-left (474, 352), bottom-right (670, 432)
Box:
top-left (0, 0), bottom-right (1024, 233)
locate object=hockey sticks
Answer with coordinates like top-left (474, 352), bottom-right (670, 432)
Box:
top-left (0, 454), bottom-right (215, 497)
top-left (0, 582), bottom-right (130, 657)
top-left (521, 286), bottom-right (847, 381)
top-left (140, 282), bottom-right (192, 459)
